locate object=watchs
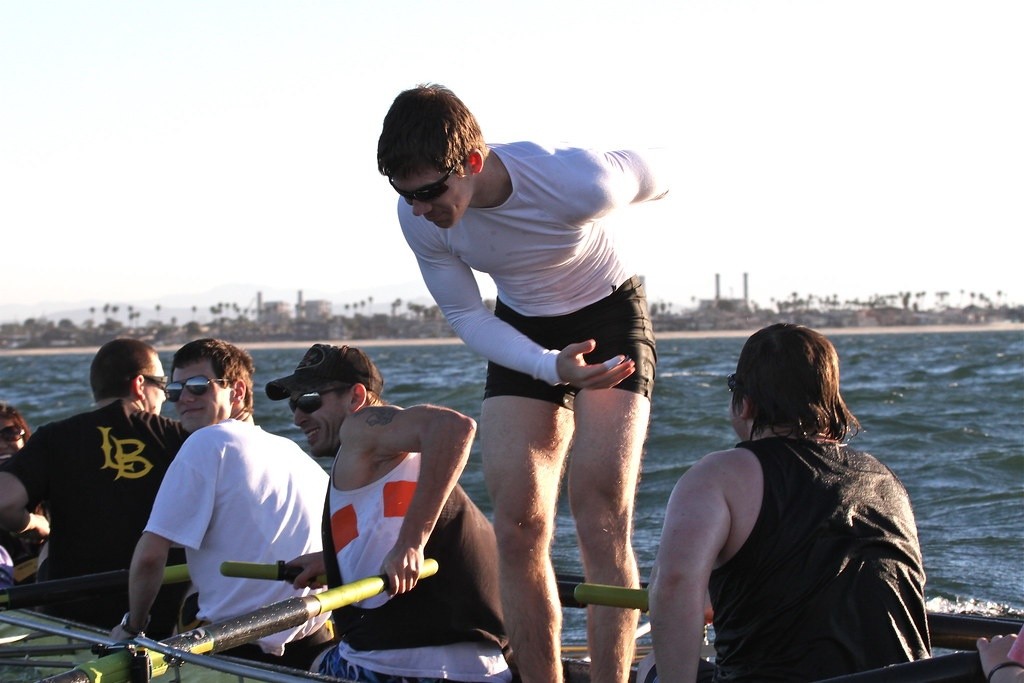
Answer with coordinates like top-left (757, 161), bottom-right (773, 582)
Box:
top-left (121, 612), bottom-right (151, 634)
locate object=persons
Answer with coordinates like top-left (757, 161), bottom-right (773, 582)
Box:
top-left (648, 321), bottom-right (930, 683)
top-left (0, 337), bottom-right (192, 641)
top-left (265, 344), bottom-right (514, 683)
top-left (977, 633), bottom-right (1024, 683)
top-left (109, 342), bottom-right (335, 670)
top-left (378, 85), bottom-right (672, 683)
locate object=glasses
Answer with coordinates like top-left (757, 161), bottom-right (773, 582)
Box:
top-left (0, 425), bottom-right (26, 444)
top-left (139, 375), bottom-right (169, 392)
top-left (727, 372), bottom-right (742, 400)
top-left (285, 384), bottom-right (344, 415)
top-left (165, 375), bottom-right (228, 402)
top-left (390, 159), bottom-right (457, 206)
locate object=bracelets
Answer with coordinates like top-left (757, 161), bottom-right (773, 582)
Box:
top-left (986, 661), bottom-right (1024, 683)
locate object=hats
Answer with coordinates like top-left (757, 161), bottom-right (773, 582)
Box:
top-left (265, 342), bottom-right (383, 401)
